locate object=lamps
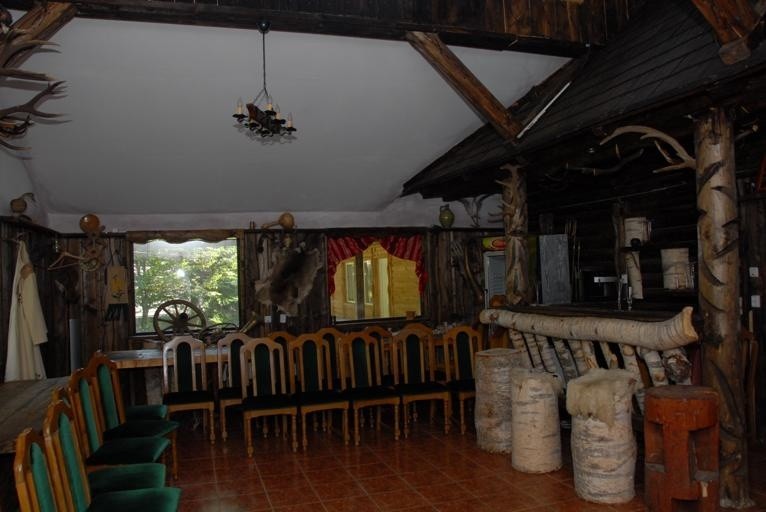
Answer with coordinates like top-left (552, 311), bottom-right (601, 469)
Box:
top-left (231, 20), bottom-right (298, 138)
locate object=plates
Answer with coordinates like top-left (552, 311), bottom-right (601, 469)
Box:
top-left (79, 215), bottom-right (101, 234)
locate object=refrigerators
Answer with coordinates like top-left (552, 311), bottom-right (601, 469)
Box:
top-left (483, 233), bottom-right (574, 348)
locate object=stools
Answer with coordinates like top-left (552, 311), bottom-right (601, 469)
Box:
top-left (642, 385), bottom-right (721, 511)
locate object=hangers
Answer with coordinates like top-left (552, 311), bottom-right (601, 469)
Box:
top-left (47, 242), bottom-right (89, 271)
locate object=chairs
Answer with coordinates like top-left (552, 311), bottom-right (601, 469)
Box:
top-left (12, 323), bottom-right (177, 512)
top-left (161, 321), bottom-right (484, 459)
top-left (741, 324), bottom-right (759, 447)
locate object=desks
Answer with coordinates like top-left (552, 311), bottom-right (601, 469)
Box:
top-left (1, 376), bottom-right (71, 456)
